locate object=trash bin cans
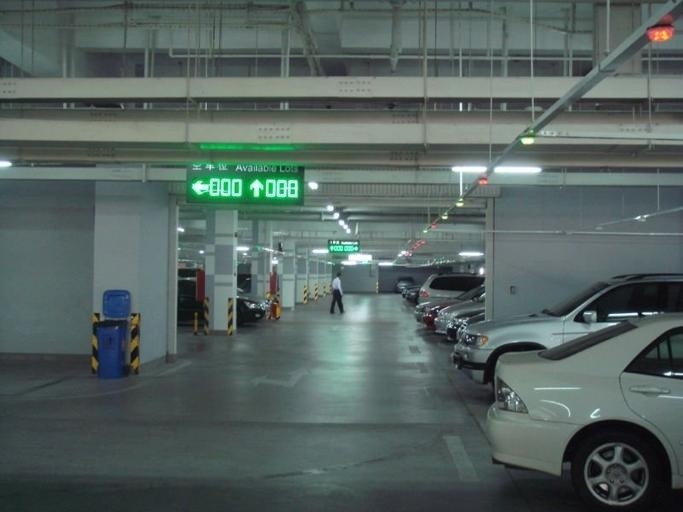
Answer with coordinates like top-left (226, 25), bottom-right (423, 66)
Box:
top-left (93, 290), bottom-right (130, 379)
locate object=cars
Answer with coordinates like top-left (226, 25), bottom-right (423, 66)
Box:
top-left (178, 277), bottom-right (265, 323)
top-left (482, 314), bottom-right (683, 512)
top-left (396, 277), bottom-right (414, 292)
top-left (402, 273), bottom-right (485, 340)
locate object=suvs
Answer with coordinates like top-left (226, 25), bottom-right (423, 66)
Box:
top-left (450, 271), bottom-right (683, 392)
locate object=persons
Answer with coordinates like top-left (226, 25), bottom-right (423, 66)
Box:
top-left (329, 271), bottom-right (344, 313)
